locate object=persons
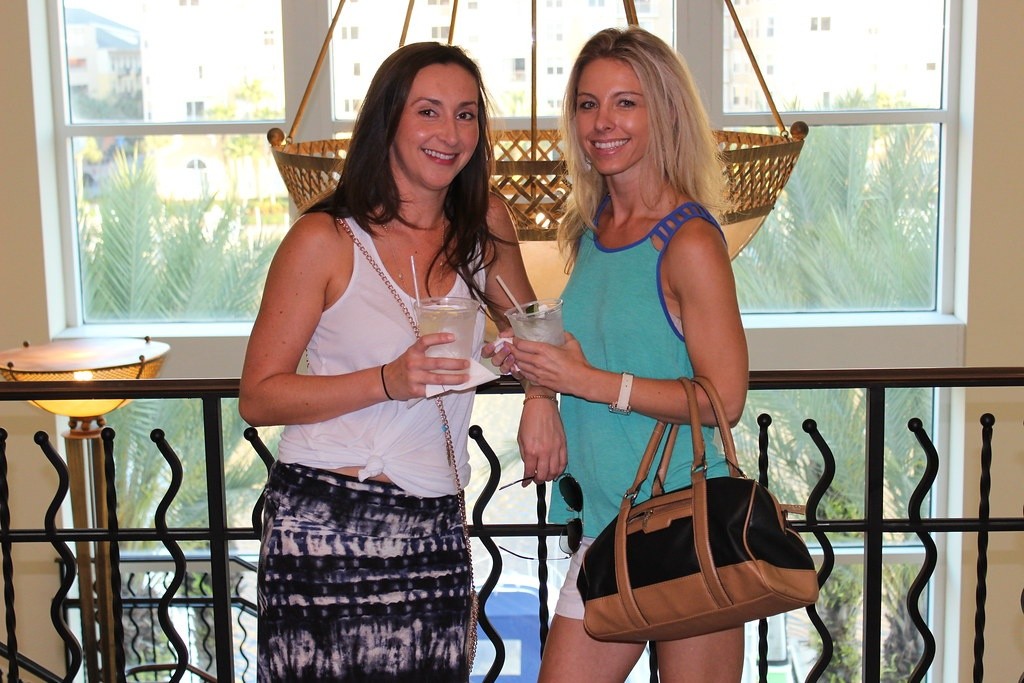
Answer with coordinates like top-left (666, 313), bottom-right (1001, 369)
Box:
top-left (479, 26), bottom-right (750, 683)
top-left (238, 44), bottom-right (568, 683)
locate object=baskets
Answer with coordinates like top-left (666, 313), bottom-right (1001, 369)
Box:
top-left (268, 0), bottom-right (809, 344)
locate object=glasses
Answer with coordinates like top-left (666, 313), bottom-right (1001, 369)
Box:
top-left (499, 473), bottom-right (583, 560)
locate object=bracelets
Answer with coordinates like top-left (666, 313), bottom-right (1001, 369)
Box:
top-left (610, 372), bottom-right (634, 415)
top-left (523, 395), bottom-right (557, 405)
top-left (381, 364), bottom-right (394, 401)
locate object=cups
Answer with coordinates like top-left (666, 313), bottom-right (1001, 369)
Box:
top-left (503, 299), bottom-right (566, 348)
top-left (413, 296), bottom-right (480, 375)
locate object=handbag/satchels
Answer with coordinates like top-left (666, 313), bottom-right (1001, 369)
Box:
top-left (465, 593), bottom-right (478, 674)
top-left (576, 377), bottom-right (819, 644)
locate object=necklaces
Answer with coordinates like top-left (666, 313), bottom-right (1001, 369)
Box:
top-left (381, 223), bottom-right (445, 293)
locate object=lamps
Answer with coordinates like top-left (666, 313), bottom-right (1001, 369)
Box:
top-left (266, 0), bottom-right (810, 376)
top-left (0, 336), bottom-right (172, 683)
